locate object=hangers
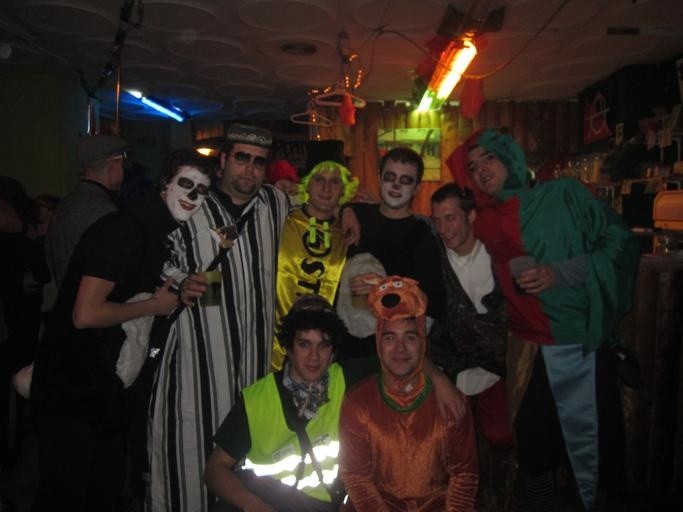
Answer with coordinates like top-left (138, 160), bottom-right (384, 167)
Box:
top-left (290, 101), bottom-right (333, 127)
top-left (315, 80), bottom-right (366, 108)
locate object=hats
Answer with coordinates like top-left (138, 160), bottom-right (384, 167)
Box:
top-left (291, 294), bottom-right (335, 313)
top-left (75, 134), bottom-right (130, 163)
top-left (227, 123), bottom-right (273, 148)
top-left (296, 140), bottom-right (352, 178)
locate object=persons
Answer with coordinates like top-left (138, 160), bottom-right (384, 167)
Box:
top-left (429, 183), bottom-right (524, 512)
top-left (265, 143), bottom-right (361, 379)
top-left (27, 190), bottom-right (62, 244)
top-left (197, 292), bottom-right (472, 512)
top-left (440, 126), bottom-right (644, 512)
top-left (138, 122), bottom-right (363, 510)
top-left (1, 170), bottom-right (40, 474)
top-left (334, 146), bottom-right (452, 379)
top-left (0, 149), bottom-right (217, 511)
top-left (43, 134), bottom-right (127, 297)
top-left (335, 272), bottom-right (482, 510)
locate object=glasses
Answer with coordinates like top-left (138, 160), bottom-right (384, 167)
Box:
top-left (110, 151), bottom-right (127, 160)
top-left (234, 151), bottom-right (267, 171)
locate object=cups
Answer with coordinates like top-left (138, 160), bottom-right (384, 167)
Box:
top-left (195, 271), bottom-right (222, 308)
top-left (349, 279), bottom-right (369, 309)
top-left (652, 234), bottom-right (672, 257)
top-left (508, 255), bottom-right (535, 296)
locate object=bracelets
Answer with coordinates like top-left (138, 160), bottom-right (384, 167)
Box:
top-left (338, 203), bottom-right (348, 220)
